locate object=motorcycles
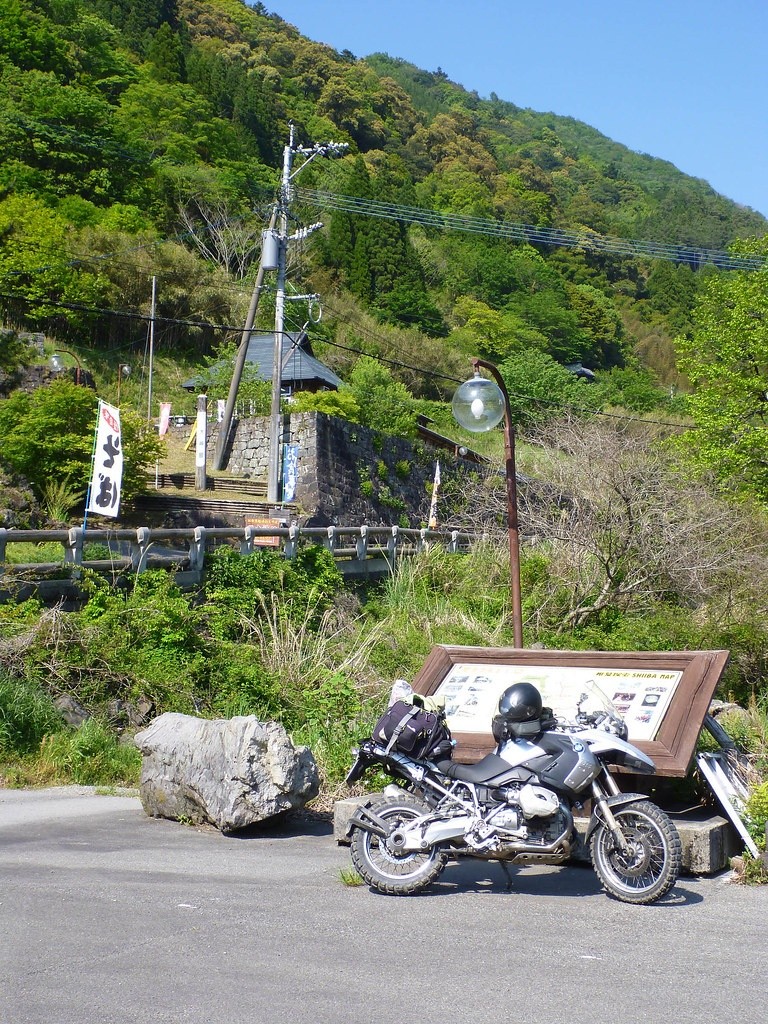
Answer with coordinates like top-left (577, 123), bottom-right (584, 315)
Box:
top-left (339, 682), bottom-right (683, 907)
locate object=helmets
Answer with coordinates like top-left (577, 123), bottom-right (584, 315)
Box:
top-left (499, 683), bottom-right (542, 722)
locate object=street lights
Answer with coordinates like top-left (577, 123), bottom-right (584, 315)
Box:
top-left (49, 348), bottom-right (81, 386)
top-left (450, 355), bottom-right (524, 649)
top-left (117, 363), bottom-right (132, 408)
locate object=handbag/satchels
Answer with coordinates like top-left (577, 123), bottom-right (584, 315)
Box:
top-left (372, 699), bottom-right (450, 760)
